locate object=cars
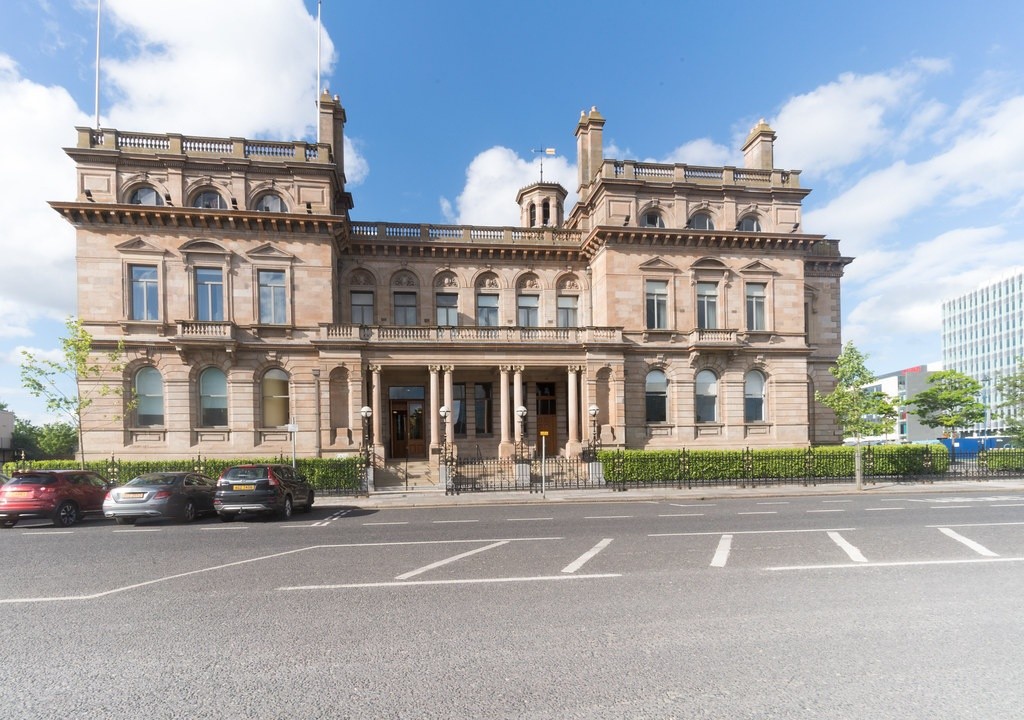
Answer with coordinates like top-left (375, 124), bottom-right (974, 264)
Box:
top-left (213, 464), bottom-right (314, 522)
top-left (102, 472), bottom-right (217, 525)
top-left (0, 469), bottom-right (117, 528)
